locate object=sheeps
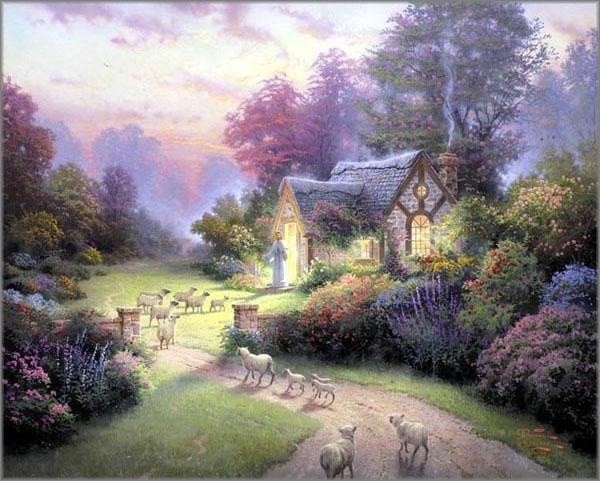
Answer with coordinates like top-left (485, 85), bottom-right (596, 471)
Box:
top-left (236, 344), bottom-right (335, 405)
top-left (138, 285), bottom-right (229, 351)
top-left (319, 424), bottom-right (358, 481)
top-left (386, 412), bottom-right (429, 462)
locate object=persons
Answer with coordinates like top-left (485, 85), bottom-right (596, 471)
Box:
top-left (261, 231), bottom-right (289, 288)
